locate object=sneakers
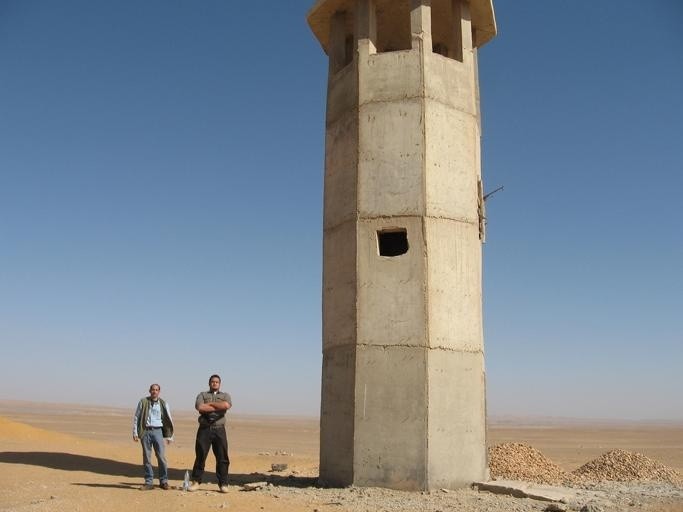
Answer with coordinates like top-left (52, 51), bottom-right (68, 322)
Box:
top-left (140, 484), bottom-right (153, 489)
top-left (220, 485), bottom-right (228, 493)
top-left (187, 481), bottom-right (199, 491)
top-left (162, 483), bottom-right (169, 490)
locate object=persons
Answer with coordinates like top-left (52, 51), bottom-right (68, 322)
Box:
top-left (187, 374), bottom-right (231, 494)
top-left (131, 383), bottom-right (175, 491)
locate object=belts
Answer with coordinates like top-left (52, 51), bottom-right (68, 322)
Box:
top-left (144, 427), bottom-right (162, 430)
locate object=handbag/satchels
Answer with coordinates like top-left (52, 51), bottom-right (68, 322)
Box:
top-left (203, 415), bottom-right (218, 424)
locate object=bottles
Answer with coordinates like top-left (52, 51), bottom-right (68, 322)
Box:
top-left (182, 470), bottom-right (190, 490)
